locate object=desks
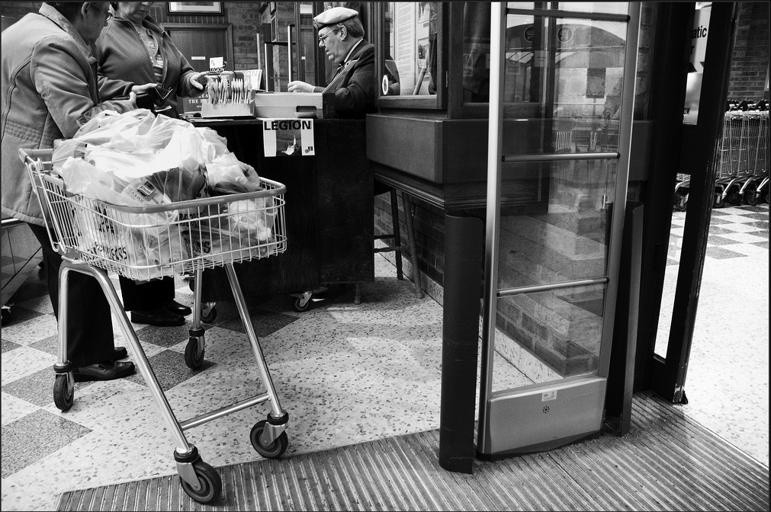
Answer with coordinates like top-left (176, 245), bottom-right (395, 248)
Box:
top-left (178, 111), bottom-right (375, 306)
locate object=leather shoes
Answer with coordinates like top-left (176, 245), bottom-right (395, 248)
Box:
top-left (132, 309), bottom-right (184, 326)
top-left (72, 362), bottom-right (135, 382)
top-left (108, 347), bottom-right (126, 360)
top-left (170, 300), bottom-right (191, 316)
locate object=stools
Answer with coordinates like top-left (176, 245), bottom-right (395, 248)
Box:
top-left (353, 177), bottom-right (426, 305)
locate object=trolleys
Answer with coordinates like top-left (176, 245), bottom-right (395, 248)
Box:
top-left (18, 146), bottom-right (289, 505)
top-left (552, 103), bottom-right (622, 228)
top-left (672, 99), bottom-right (771, 213)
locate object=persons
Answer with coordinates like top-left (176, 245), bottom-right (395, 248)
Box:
top-left (0, 1), bottom-right (137, 384)
top-left (92, 1), bottom-right (210, 328)
top-left (286, 6), bottom-right (376, 121)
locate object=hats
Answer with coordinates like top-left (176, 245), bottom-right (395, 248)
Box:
top-left (313, 7), bottom-right (358, 31)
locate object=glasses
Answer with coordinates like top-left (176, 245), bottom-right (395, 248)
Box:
top-left (91, 5), bottom-right (110, 21)
top-left (319, 29), bottom-right (335, 43)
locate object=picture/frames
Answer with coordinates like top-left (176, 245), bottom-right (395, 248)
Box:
top-left (167, 0), bottom-right (226, 16)
